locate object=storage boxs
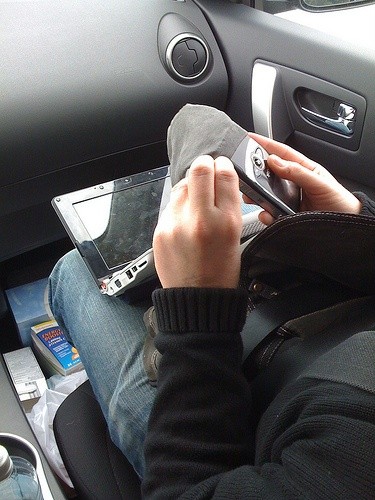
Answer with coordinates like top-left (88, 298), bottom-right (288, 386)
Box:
top-left (4, 278), bottom-right (51, 347)
top-left (2, 347), bottom-right (48, 414)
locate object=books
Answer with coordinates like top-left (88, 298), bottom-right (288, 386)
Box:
top-left (29, 323), bottom-right (83, 375)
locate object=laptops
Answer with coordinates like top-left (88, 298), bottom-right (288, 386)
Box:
top-left (50, 164), bottom-right (267, 298)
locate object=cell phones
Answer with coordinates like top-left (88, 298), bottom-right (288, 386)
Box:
top-left (215, 124), bottom-right (302, 219)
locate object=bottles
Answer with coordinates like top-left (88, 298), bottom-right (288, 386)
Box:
top-left (0, 446), bottom-right (43, 499)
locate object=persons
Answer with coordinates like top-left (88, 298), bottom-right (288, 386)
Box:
top-left (49, 132), bottom-right (375, 500)
